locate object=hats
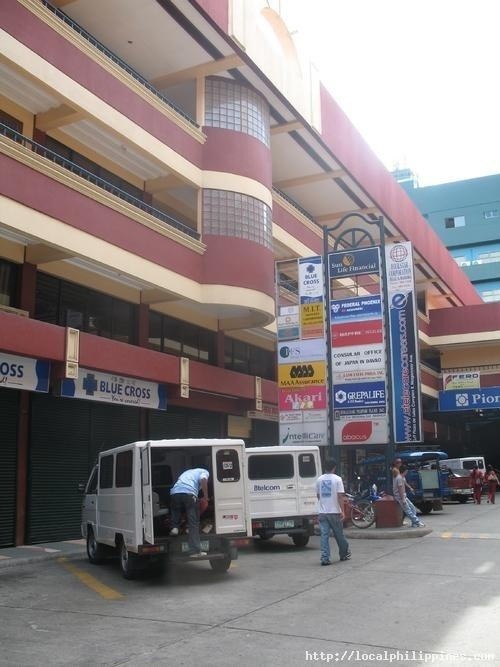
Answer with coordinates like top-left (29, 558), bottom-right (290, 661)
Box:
top-left (442, 465), bottom-right (485, 504)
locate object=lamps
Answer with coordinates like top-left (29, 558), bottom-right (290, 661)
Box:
top-left (152, 491), bottom-right (168, 517)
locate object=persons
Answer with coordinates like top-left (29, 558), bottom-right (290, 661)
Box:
top-left (169, 468), bottom-right (210, 558)
top-left (392, 465), bottom-right (425, 528)
top-left (313, 457), bottom-right (351, 565)
top-left (392, 458), bottom-right (422, 514)
top-left (471, 464), bottom-right (500, 504)
